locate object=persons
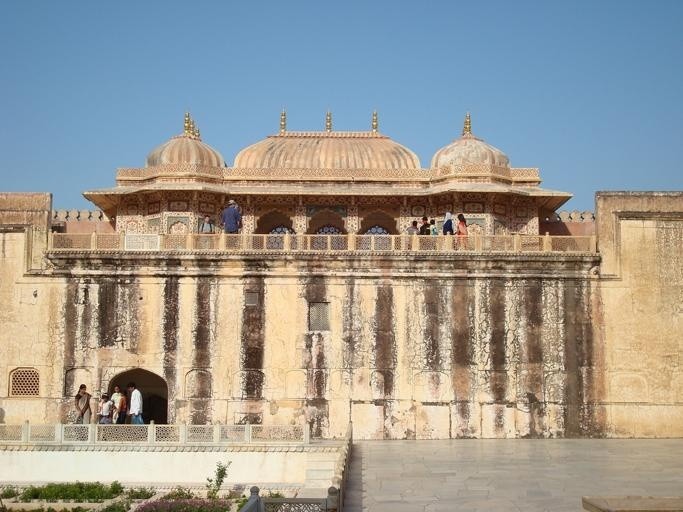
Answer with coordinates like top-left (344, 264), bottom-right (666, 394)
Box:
top-left (430, 220), bottom-right (438, 235)
top-left (127, 381), bottom-right (144, 424)
top-left (221, 199), bottom-right (241, 234)
top-left (406, 220), bottom-right (420, 235)
top-left (455, 214), bottom-right (468, 234)
top-left (97, 393), bottom-right (116, 424)
top-left (199, 215), bottom-right (215, 234)
top-left (111, 385), bottom-right (127, 424)
top-left (442, 212), bottom-right (455, 234)
top-left (75, 384), bottom-right (91, 423)
top-left (419, 216), bottom-right (430, 235)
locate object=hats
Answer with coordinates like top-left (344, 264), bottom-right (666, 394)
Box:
top-left (228, 200), bottom-right (236, 204)
